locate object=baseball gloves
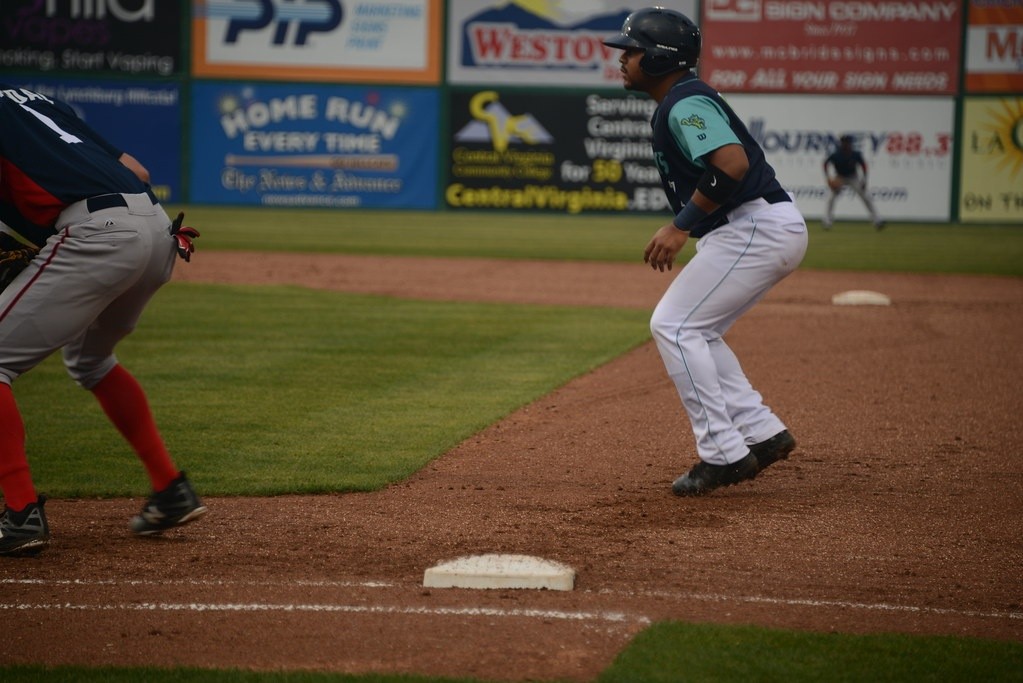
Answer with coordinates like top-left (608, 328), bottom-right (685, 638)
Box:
top-left (0, 229), bottom-right (41, 297)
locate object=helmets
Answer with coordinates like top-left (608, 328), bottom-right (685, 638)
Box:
top-left (602, 7), bottom-right (701, 77)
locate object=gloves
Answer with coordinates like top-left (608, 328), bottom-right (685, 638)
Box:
top-left (171, 212), bottom-right (200, 263)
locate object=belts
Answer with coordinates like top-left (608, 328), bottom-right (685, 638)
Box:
top-left (696, 191), bottom-right (792, 238)
top-left (46, 190), bottom-right (159, 237)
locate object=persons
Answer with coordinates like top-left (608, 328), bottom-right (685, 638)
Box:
top-left (600, 7), bottom-right (809, 497)
top-left (822, 135), bottom-right (883, 230)
top-left (0, 85), bottom-right (210, 557)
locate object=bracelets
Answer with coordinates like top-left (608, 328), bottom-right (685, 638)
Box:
top-left (673, 200), bottom-right (709, 234)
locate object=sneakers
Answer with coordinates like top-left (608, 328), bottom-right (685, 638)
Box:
top-left (672, 450), bottom-right (760, 497)
top-left (0, 495), bottom-right (49, 556)
top-left (746, 429), bottom-right (796, 471)
top-left (128, 471), bottom-right (207, 536)
